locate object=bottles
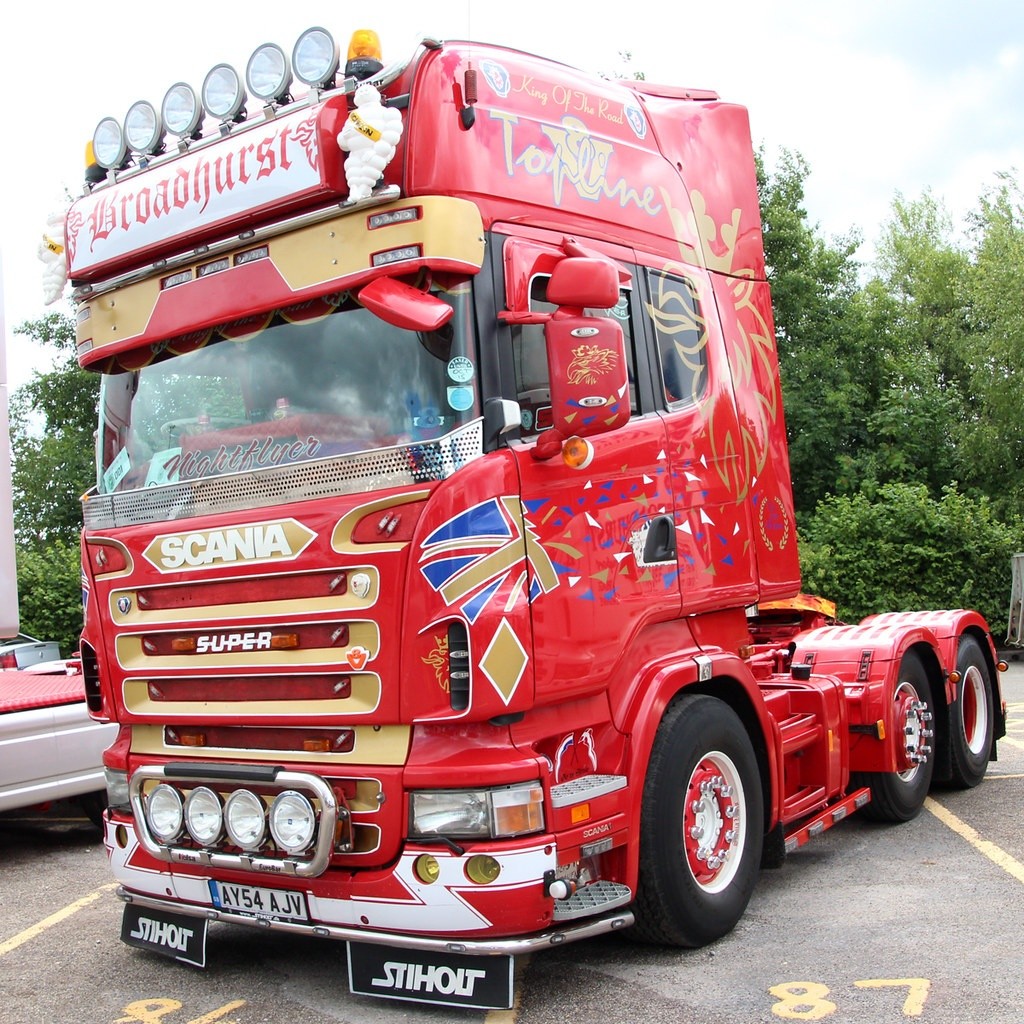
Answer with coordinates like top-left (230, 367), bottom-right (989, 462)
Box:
top-left (196, 416), bottom-right (216, 434)
top-left (273, 396), bottom-right (295, 420)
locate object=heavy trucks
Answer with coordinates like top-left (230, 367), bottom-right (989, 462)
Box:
top-left (56, 20), bottom-right (1010, 1014)
